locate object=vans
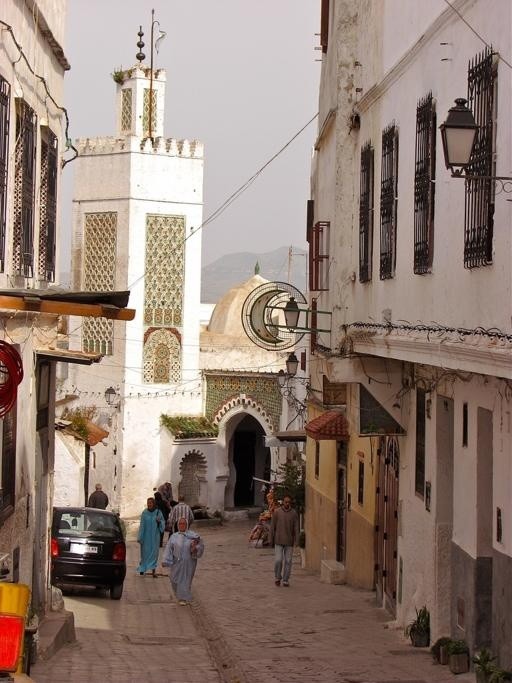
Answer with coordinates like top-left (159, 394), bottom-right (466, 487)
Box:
top-left (53, 505), bottom-right (127, 600)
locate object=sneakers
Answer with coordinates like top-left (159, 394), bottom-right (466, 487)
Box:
top-left (178, 599), bottom-right (187, 606)
top-left (139, 571), bottom-right (158, 578)
top-left (274, 581), bottom-right (289, 587)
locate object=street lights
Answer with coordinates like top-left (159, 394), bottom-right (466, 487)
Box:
top-left (437, 94), bottom-right (512, 212)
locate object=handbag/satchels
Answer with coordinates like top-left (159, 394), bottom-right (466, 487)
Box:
top-left (159, 529), bottom-right (164, 548)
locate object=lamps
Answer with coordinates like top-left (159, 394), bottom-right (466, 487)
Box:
top-left (283, 297), bottom-right (317, 354)
top-left (438, 97), bottom-right (512, 197)
top-left (286, 352), bottom-right (311, 386)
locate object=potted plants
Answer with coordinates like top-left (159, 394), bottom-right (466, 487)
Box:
top-left (430, 636), bottom-right (470, 674)
top-left (470, 646), bottom-right (512, 683)
top-left (404, 603), bottom-right (430, 647)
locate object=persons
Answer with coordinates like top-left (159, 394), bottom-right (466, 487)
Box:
top-left (267, 495), bottom-right (301, 586)
top-left (166, 494), bottom-right (194, 535)
top-left (87, 483), bottom-right (109, 510)
top-left (137, 497), bottom-right (166, 578)
top-left (161, 518), bottom-right (205, 605)
top-left (152, 492), bottom-right (170, 547)
